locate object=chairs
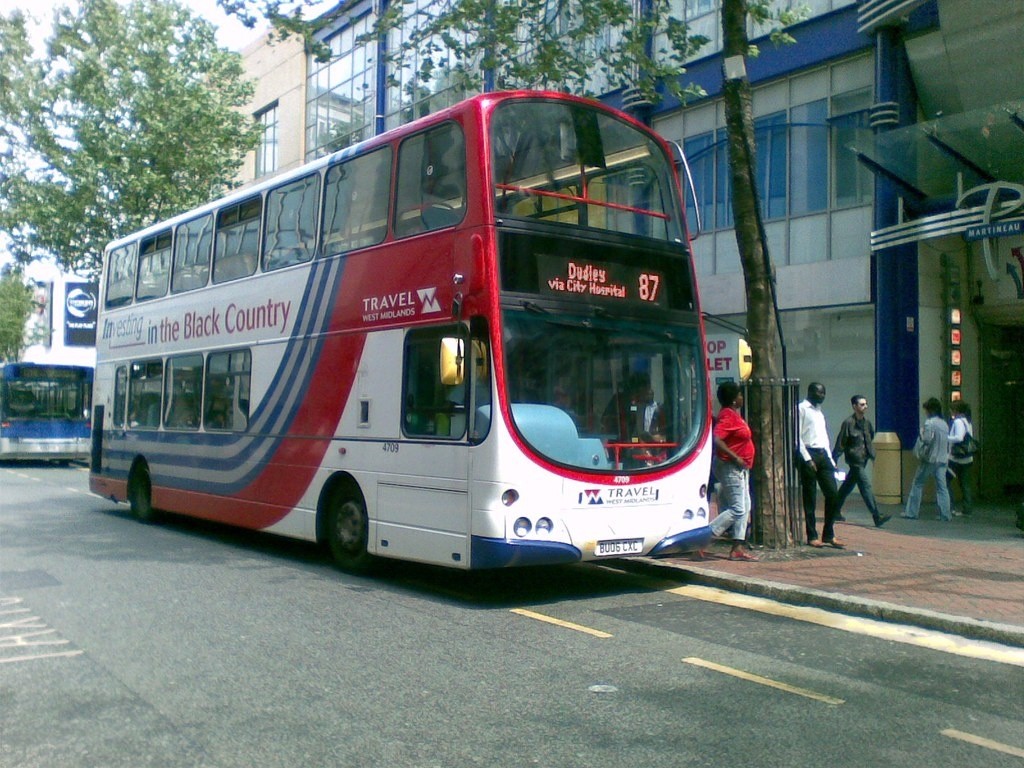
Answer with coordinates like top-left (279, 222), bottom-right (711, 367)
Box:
top-left (146, 401), bottom-right (160, 427)
top-left (108, 202), bottom-right (460, 306)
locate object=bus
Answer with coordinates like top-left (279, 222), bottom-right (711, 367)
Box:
top-left (86, 88), bottom-right (753, 580)
top-left (1, 362), bottom-right (95, 465)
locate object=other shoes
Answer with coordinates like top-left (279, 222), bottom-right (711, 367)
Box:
top-left (951, 510), bottom-right (971, 517)
top-left (728, 549), bottom-right (758, 561)
top-left (697, 549), bottom-right (704, 559)
top-left (827, 538), bottom-right (848, 546)
top-left (835, 514), bottom-right (846, 521)
top-left (900, 511), bottom-right (907, 518)
top-left (808, 540), bottom-right (822, 546)
top-left (875, 514), bottom-right (891, 528)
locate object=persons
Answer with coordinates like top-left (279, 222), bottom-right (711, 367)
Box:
top-left (600, 369), bottom-right (664, 434)
top-left (832, 395), bottom-right (892, 527)
top-left (790, 383), bottom-right (847, 548)
top-left (900, 397), bottom-right (953, 522)
top-left (947, 399), bottom-right (974, 519)
top-left (694, 382), bottom-right (758, 561)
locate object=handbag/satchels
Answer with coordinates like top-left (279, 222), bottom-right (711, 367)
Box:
top-left (914, 420), bottom-right (934, 462)
top-left (950, 418), bottom-right (981, 458)
